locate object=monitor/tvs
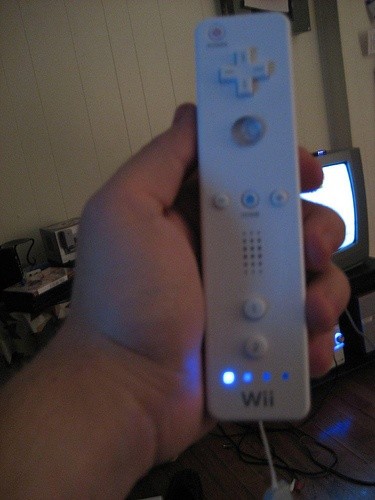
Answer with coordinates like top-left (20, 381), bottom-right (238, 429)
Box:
top-left (300, 145), bottom-right (369, 276)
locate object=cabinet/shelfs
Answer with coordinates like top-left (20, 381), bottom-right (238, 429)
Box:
top-left (0, 279), bottom-right (73, 314)
top-left (308, 256), bottom-right (375, 389)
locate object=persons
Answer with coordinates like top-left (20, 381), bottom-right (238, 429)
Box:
top-left (1, 101), bottom-right (353, 499)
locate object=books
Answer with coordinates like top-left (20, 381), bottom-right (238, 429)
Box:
top-left (3, 266), bottom-right (76, 296)
